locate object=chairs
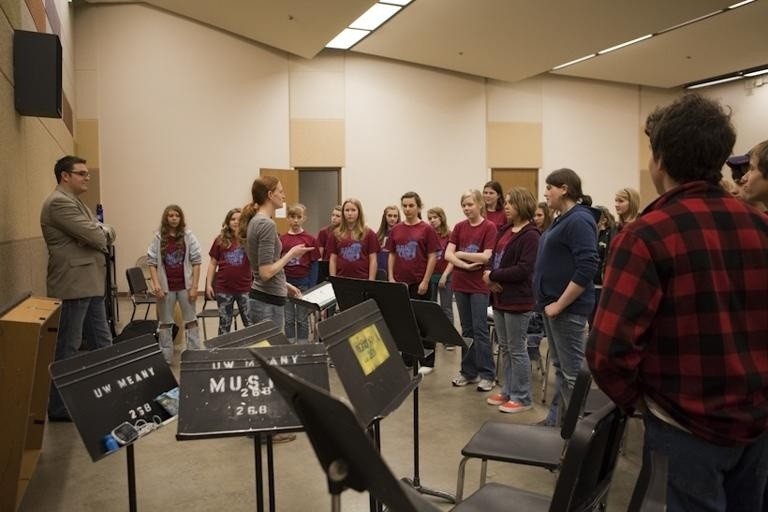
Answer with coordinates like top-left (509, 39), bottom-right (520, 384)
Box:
top-left (450, 401), bottom-right (626, 512)
top-left (124, 267), bottom-right (172, 327)
top-left (446, 312), bottom-right (552, 404)
top-left (194, 273), bottom-right (242, 345)
top-left (455, 358), bottom-right (593, 504)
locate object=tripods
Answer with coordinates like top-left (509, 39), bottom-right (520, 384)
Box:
top-left (382, 342), bottom-right (457, 512)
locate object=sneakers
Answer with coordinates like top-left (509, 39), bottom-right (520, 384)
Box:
top-left (499, 398), bottom-right (533, 413)
top-left (475, 378), bottom-right (497, 393)
top-left (418, 366), bottom-right (433, 376)
top-left (485, 392), bottom-right (510, 405)
top-left (451, 371), bottom-right (482, 385)
top-left (269, 432), bottom-right (297, 443)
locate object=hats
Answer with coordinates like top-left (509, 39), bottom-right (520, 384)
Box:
top-left (726, 147), bottom-right (753, 168)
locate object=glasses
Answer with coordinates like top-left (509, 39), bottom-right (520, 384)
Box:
top-left (71, 171), bottom-right (91, 177)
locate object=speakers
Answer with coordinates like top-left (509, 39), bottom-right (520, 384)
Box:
top-left (13, 28), bottom-right (62, 118)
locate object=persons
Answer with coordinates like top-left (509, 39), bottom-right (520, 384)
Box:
top-left (318, 206), bottom-right (343, 261)
top-left (531, 167), bottom-right (599, 394)
top-left (479, 181), bottom-right (507, 232)
top-left (280, 204), bottom-right (321, 344)
top-left (327, 198), bottom-right (381, 279)
top-left (236, 176), bottom-right (315, 444)
top-left (206, 208), bottom-right (253, 337)
top-left (482, 187), bottom-right (543, 413)
top-left (727, 140), bottom-right (766, 216)
top-left (589, 187), bottom-right (641, 317)
top-left (427, 207), bottom-right (455, 351)
top-left (374, 204), bottom-right (401, 269)
top-left (384, 191), bottom-right (443, 375)
top-left (585, 93), bottom-right (766, 510)
top-left (40, 155), bottom-right (116, 421)
top-left (526, 200), bottom-right (553, 367)
top-left (444, 188), bottom-right (498, 391)
top-left (145, 206), bottom-right (201, 366)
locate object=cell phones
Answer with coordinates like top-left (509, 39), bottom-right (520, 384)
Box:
top-left (477, 264), bottom-right (485, 269)
